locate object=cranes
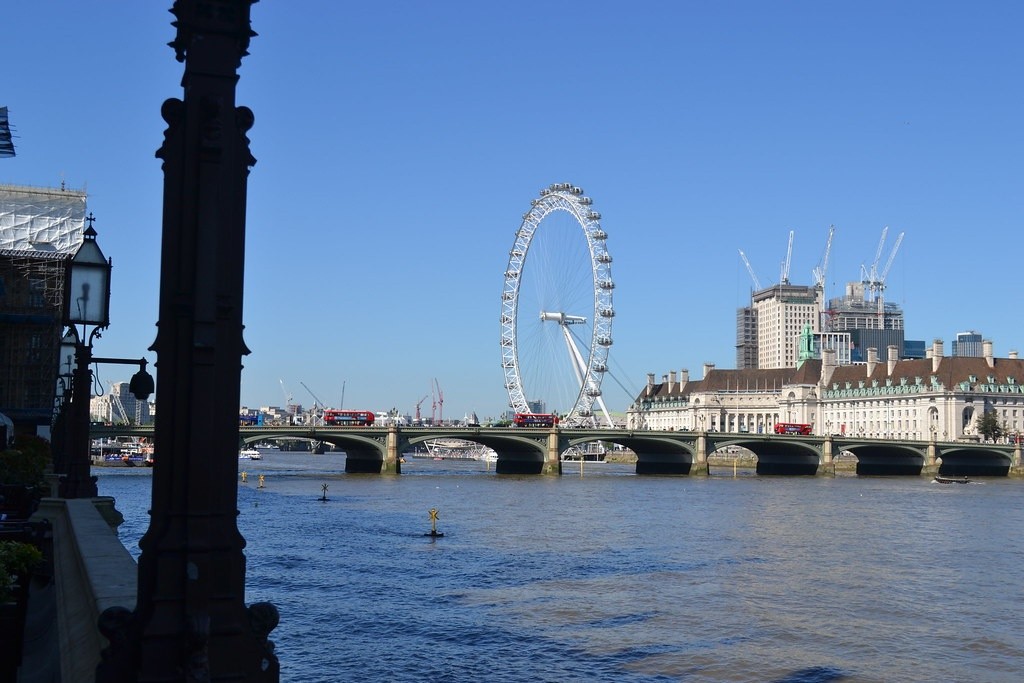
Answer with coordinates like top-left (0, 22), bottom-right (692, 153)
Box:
top-left (416, 395), bottom-right (426, 421)
top-left (860, 226), bottom-right (889, 300)
top-left (737, 249), bottom-right (762, 292)
top-left (872, 232), bottom-right (905, 329)
top-left (279, 379), bottom-right (292, 413)
top-left (813, 223), bottom-right (836, 331)
top-left (782, 229), bottom-right (795, 285)
top-left (431, 379), bottom-right (437, 425)
top-left (435, 378), bottom-right (445, 426)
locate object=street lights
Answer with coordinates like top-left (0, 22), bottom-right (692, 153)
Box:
top-left (51, 212), bottom-right (111, 497)
top-left (501, 413), bottom-right (507, 426)
top-left (943, 430), bottom-right (947, 441)
top-left (826, 420), bottom-right (831, 436)
top-left (700, 414), bottom-right (706, 431)
top-left (859, 427), bottom-right (864, 437)
top-left (930, 426), bottom-right (935, 440)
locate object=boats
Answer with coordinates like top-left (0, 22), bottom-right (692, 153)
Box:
top-left (935, 475), bottom-right (972, 485)
top-left (241, 449), bottom-right (262, 460)
top-left (93, 442), bottom-right (153, 468)
top-left (400, 458), bottom-right (407, 463)
top-left (434, 457), bottom-right (444, 461)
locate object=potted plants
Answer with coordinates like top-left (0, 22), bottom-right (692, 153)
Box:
top-left (0, 449), bottom-right (40, 520)
top-left (0, 538), bottom-right (48, 683)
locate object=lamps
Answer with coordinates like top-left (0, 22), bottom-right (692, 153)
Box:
top-left (48, 325), bottom-right (81, 495)
top-left (63, 211), bottom-right (110, 499)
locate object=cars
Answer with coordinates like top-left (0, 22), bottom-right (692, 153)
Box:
top-left (679, 429), bottom-right (690, 431)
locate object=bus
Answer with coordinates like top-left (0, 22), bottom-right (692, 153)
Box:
top-left (324, 410), bottom-right (375, 426)
top-left (775, 423), bottom-right (812, 435)
top-left (1014, 432), bottom-right (1024, 445)
top-left (514, 413), bottom-right (559, 427)
